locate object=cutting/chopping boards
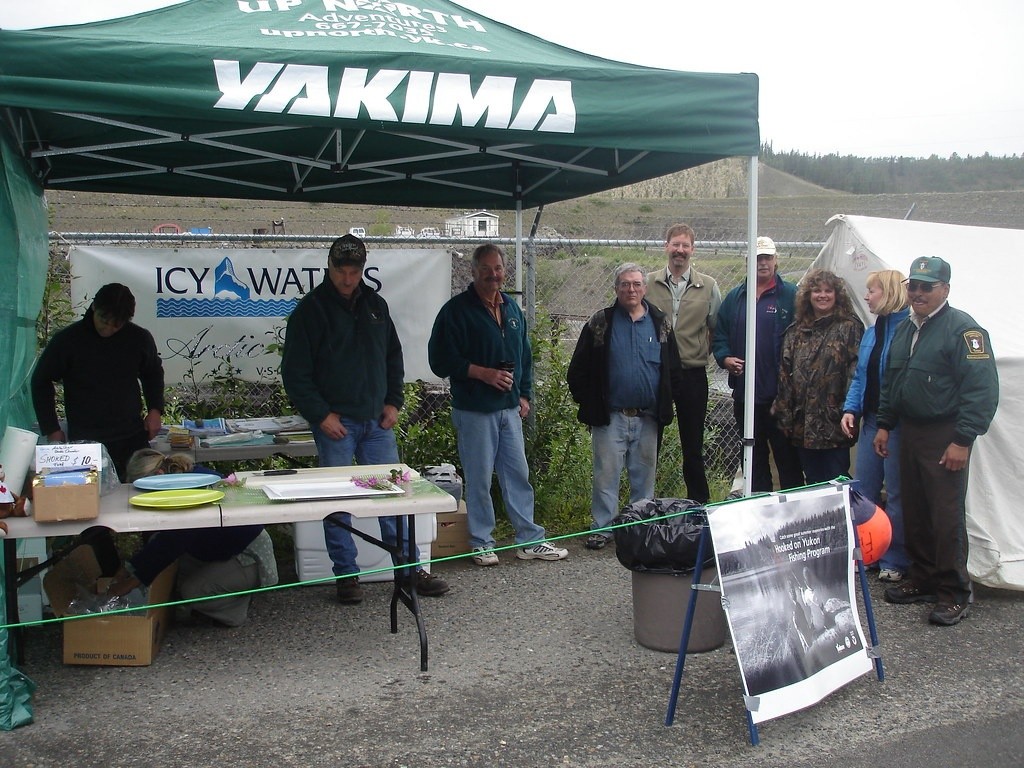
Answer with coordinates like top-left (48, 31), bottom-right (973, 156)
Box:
top-left (234, 463), bottom-right (419, 486)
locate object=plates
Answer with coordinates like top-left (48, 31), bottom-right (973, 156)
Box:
top-left (261, 480), bottom-right (405, 502)
top-left (133, 473), bottom-right (222, 491)
top-left (129, 489), bottom-right (225, 511)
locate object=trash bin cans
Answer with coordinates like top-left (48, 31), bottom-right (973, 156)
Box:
top-left (628, 499), bottom-right (726, 652)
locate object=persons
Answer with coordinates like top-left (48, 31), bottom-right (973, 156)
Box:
top-left (774, 268), bottom-right (865, 485)
top-left (29, 281), bottom-right (167, 580)
top-left (871, 256), bottom-right (1000, 627)
top-left (106, 446), bottom-right (279, 629)
top-left (709, 234), bottom-right (806, 495)
top-left (839, 268), bottom-right (913, 583)
top-left (643, 221), bottom-right (722, 509)
top-left (428, 243), bottom-right (569, 567)
top-left (281, 233), bottom-right (452, 607)
top-left (566, 261), bottom-right (685, 550)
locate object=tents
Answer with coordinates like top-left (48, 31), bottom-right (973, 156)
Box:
top-left (793, 213), bottom-right (1024, 594)
top-left (0, 0), bottom-right (762, 665)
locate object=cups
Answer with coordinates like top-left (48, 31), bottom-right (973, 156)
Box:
top-left (496, 360), bottom-right (514, 393)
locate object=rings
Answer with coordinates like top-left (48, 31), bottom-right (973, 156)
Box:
top-left (734, 370), bottom-right (738, 374)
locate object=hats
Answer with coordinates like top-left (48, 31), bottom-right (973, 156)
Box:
top-left (328, 234), bottom-right (367, 266)
top-left (745, 236), bottom-right (776, 258)
top-left (901, 255), bottom-right (952, 283)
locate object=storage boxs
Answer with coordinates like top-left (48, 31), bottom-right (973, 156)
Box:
top-left (33, 470), bottom-right (99, 522)
top-left (293, 512), bottom-right (437, 585)
top-left (43, 545), bottom-right (180, 665)
top-left (431, 499), bottom-right (471, 558)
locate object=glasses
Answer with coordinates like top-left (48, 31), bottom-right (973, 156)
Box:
top-left (757, 255), bottom-right (774, 261)
top-left (904, 282), bottom-right (943, 292)
top-left (620, 281), bottom-right (642, 289)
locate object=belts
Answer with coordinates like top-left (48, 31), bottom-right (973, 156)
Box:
top-left (614, 407), bottom-right (648, 417)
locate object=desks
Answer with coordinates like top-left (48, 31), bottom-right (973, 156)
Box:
top-left (149, 433), bottom-right (319, 469)
top-left (0, 464), bottom-right (458, 671)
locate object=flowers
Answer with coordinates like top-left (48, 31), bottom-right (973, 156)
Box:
top-left (219, 473), bottom-right (246, 489)
top-left (350, 475), bottom-right (396, 491)
top-left (387, 467), bottom-right (410, 485)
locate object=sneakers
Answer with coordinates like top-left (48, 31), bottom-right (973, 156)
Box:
top-left (395, 570), bottom-right (450, 596)
top-left (928, 600), bottom-right (970, 625)
top-left (855, 565), bottom-right (870, 572)
top-left (879, 568), bottom-right (902, 582)
top-left (586, 534), bottom-right (610, 549)
top-left (885, 582), bottom-right (936, 604)
top-left (516, 542), bottom-right (569, 560)
top-left (337, 577), bottom-right (365, 602)
top-left (472, 546), bottom-right (499, 566)
